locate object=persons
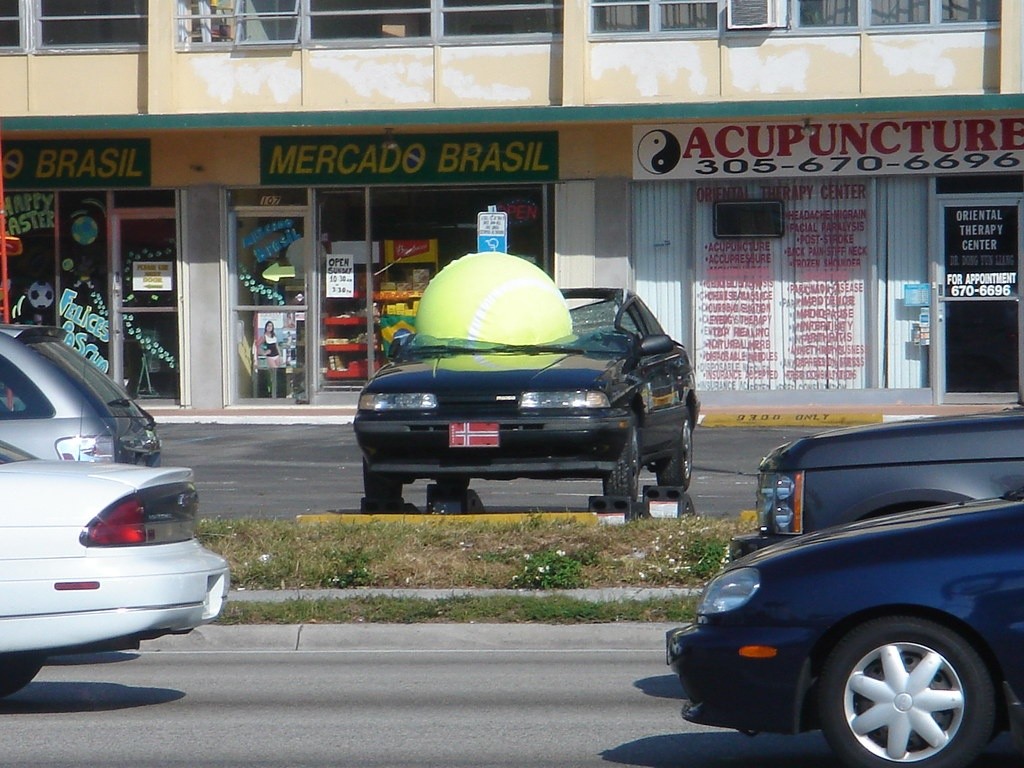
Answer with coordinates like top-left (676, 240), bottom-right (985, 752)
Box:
top-left (255, 313), bottom-right (296, 368)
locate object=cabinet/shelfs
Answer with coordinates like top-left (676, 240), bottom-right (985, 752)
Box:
top-left (322, 290), bottom-right (381, 379)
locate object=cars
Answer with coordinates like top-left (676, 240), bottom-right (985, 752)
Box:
top-left (0, 438), bottom-right (232, 704)
top-left (663, 487), bottom-right (1023, 768)
top-left (350, 286), bottom-right (702, 502)
top-left (0, 322), bottom-right (162, 474)
top-left (727, 404), bottom-right (1024, 565)
